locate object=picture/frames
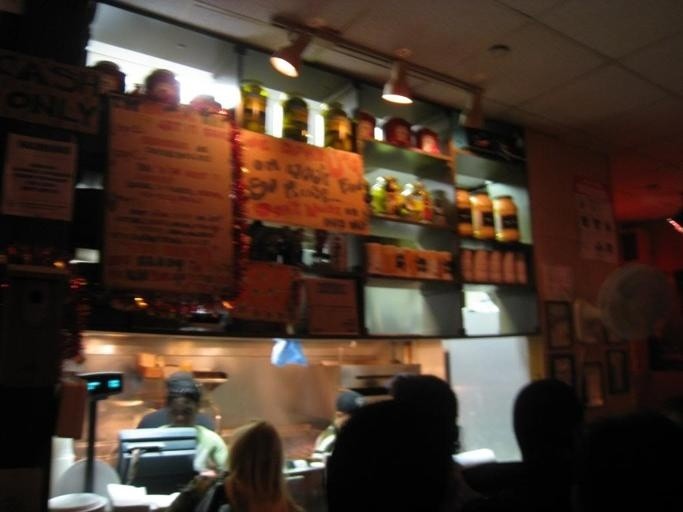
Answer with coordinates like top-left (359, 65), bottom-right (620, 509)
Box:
top-left (544, 300), bottom-right (631, 412)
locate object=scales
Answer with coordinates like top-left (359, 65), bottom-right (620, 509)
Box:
top-left (74, 371), bottom-right (124, 493)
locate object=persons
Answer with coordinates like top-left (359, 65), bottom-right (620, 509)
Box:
top-left (117, 368), bottom-right (683, 512)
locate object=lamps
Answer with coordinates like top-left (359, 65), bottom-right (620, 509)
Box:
top-left (270, 26), bottom-right (316, 78)
top-left (379, 58), bottom-right (416, 106)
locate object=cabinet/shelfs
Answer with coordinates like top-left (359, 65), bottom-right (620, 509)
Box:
top-left (453, 110), bottom-right (542, 339)
top-left (357, 86), bottom-right (463, 340)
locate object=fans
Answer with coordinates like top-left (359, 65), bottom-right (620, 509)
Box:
top-left (571, 260), bottom-right (677, 347)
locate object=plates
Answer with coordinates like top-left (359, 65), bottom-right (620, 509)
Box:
top-left (47, 492), bottom-right (107, 511)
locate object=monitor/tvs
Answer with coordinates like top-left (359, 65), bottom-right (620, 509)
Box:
top-left (118, 426), bottom-right (198, 495)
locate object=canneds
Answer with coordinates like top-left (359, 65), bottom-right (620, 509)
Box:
top-left (455, 187), bottom-right (522, 242)
